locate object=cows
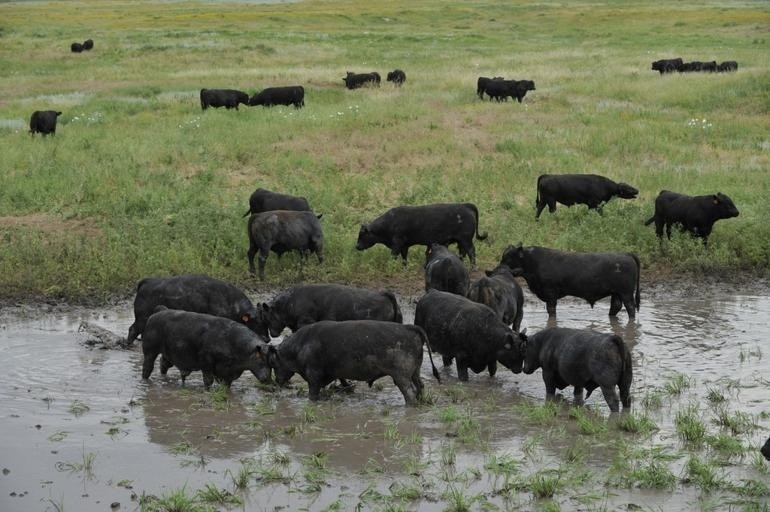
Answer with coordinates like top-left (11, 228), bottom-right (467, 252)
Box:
top-left (126, 241), bottom-right (641, 412)
top-left (355, 199), bottom-right (491, 272)
top-left (241, 187), bottom-right (326, 282)
top-left (27, 109), bottom-right (63, 138)
top-left (650, 57), bottom-right (739, 77)
top-left (475, 75), bottom-right (537, 104)
top-left (199, 85), bottom-right (305, 109)
top-left (643, 189), bottom-right (741, 248)
top-left (342, 69), bottom-right (408, 90)
top-left (533, 173), bottom-right (640, 223)
